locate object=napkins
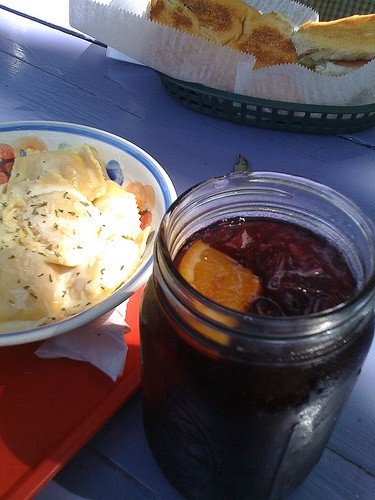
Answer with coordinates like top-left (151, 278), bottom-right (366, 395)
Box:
top-left (34, 299), bottom-right (132, 382)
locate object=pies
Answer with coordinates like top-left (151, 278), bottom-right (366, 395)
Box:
top-left (148, 0), bottom-right (375, 75)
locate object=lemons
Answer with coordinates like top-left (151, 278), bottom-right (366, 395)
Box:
top-left (179, 241), bottom-right (260, 345)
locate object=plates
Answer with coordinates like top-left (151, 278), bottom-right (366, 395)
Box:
top-left (1, 120), bottom-right (179, 347)
